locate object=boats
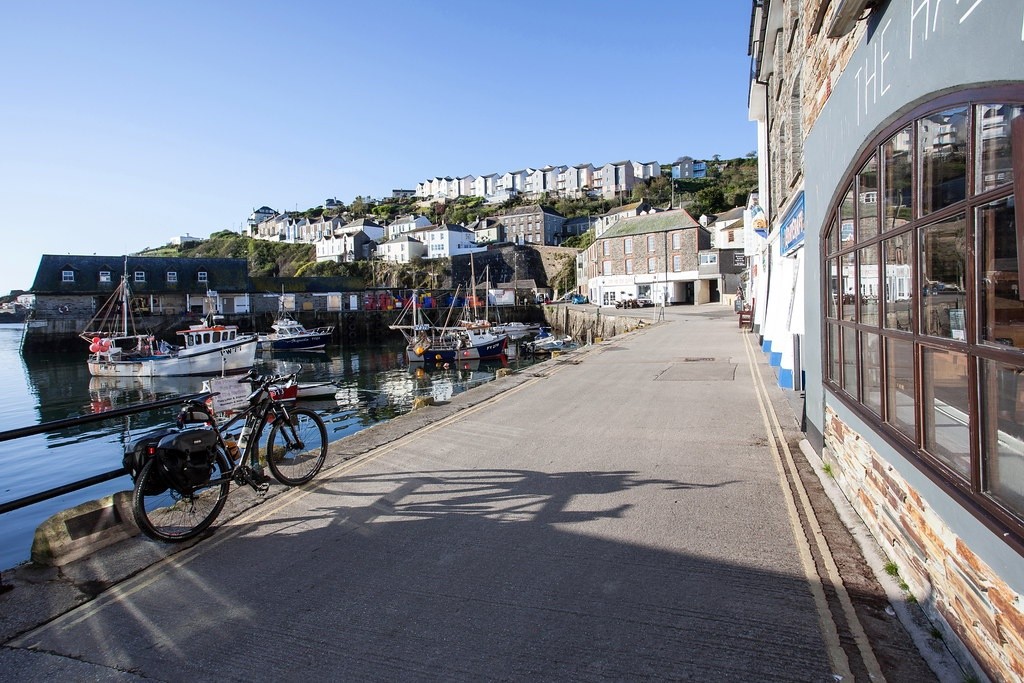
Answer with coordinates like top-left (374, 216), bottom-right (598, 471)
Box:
top-left (520, 336), bottom-right (563, 353)
top-left (476, 321), bottom-right (542, 331)
top-left (406, 357), bottom-right (501, 375)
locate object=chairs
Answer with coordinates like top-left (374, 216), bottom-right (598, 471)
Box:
top-left (737, 298), bottom-right (755, 329)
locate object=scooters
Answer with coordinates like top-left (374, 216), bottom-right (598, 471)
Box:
top-left (615, 301), bottom-right (626, 309)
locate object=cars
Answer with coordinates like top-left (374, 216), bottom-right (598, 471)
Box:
top-left (925, 283), bottom-right (945, 292)
top-left (571, 294), bottom-right (587, 304)
top-left (994, 279), bottom-right (1019, 293)
top-left (638, 299), bottom-right (655, 307)
top-left (943, 283), bottom-right (962, 292)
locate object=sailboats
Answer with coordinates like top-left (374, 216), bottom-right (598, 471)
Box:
top-left (458, 253), bottom-right (491, 329)
top-left (79, 254), bottom-right (260, 377)
top-left (388, 284), bottom-right (508, 362)
top-left (254, 283), bottom-right (335, 349)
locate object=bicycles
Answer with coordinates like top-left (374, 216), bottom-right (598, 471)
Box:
top-left (126, 362), bottom-right (328, 545)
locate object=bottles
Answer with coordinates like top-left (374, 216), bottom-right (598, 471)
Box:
top-left (223, 433), bottom-right (242, 461)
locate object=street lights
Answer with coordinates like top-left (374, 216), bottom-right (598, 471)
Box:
top-left (671, 177), bottom-right (678, 210)
top-left (617, 184), bottom-right (622, 206)
